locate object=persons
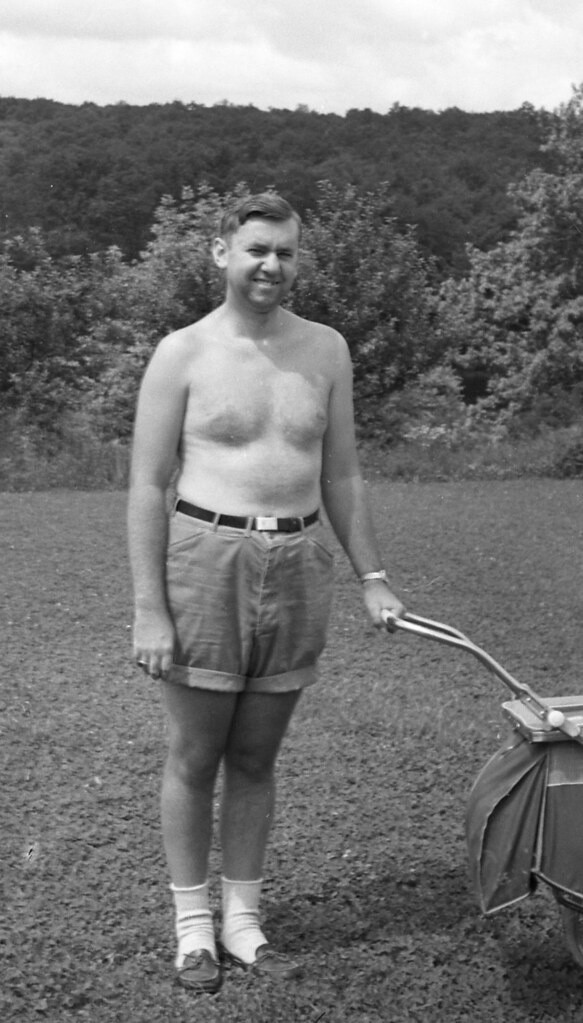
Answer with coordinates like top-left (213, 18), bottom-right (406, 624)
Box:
top-left (126, 194), bottom-right (406, 993)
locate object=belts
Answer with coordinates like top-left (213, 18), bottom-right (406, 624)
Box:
top-left (175, 498), bottom-right (319, 535)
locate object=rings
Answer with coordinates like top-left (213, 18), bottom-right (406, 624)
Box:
top-left (137, 660), bottom-right (147, 667)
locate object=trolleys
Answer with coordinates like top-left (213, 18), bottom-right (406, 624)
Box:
top-left (378, 609), bottom-right (583, 968)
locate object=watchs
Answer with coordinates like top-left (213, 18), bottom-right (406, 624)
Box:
top-left (360, 569), bottom-right (390, 587)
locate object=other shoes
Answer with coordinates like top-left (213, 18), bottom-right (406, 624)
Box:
top-left (217, 937), bottom-right (303, 980)
top-left (173, 946), bottom-right (224, 994)
